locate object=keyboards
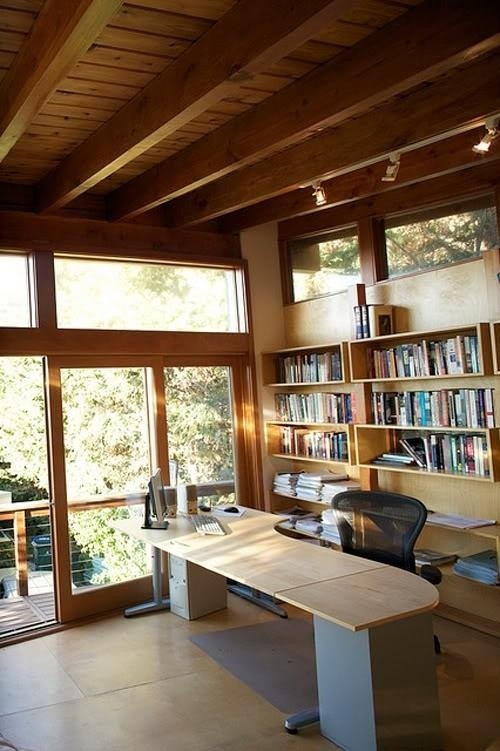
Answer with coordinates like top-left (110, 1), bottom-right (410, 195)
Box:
top-left (190, 515), bottom-right (226, 536)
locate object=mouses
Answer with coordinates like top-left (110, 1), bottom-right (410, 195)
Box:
top-left (223, 506), bottom-right (240, 512)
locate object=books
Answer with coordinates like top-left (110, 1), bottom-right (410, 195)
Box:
top-left (276, 335), bottom-right (496, 475)
top-left (428, 514), bottom-right (498, 530)
top-left (354, 304), bottom-right (393, 339)
top-left (414, 549), bottom-right (500, 584)
top-left (273, 471), bottom-right (360, 504)
top-left (273, 506), bottom-right (341, 546)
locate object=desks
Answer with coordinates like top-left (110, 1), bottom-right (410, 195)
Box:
top-left (105, 503), bottom-right (439, 751)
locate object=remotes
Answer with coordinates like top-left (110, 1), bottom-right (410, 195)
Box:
top-left (198, 505), bottom-right (212, 512)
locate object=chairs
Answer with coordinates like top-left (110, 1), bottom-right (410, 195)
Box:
top-left (331, 491), bottom-right (443, 657)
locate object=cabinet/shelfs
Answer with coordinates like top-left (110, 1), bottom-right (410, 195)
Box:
top-left (256, 320), bottom-right (499, 639)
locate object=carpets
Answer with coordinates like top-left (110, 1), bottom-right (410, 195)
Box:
top-left (187, 618), bottom-right (319, 715)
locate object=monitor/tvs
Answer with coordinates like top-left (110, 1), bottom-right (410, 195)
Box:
top-left (140, 468), bottom-right (168, 529)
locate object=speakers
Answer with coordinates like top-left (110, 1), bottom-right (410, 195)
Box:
top-left (165, 487), bottom-right (177, 517)
top-left (178, 484), bottom-right (197, 514)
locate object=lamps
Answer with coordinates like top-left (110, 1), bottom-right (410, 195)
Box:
top-left (381, 153), bottom-right (401, 182)
top-left (311, 180), bottom-right (327, 207)
top-left (471, 115), bottom-right (500, 155)
